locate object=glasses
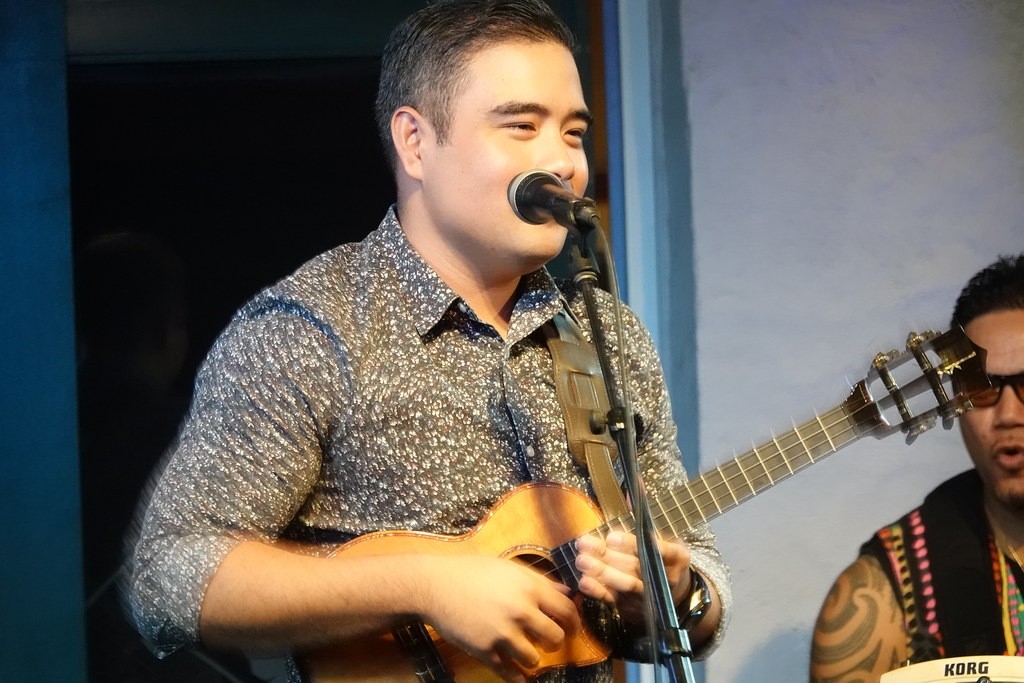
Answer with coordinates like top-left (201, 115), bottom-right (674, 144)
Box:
top-left (951, 369), bottom-right (1024, 407)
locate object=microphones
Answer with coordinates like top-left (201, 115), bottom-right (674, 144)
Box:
top-left (506, 168), bottom-right (601, 229)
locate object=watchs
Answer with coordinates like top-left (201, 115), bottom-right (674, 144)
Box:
top-left (657, 565), bottom-right (712, 631)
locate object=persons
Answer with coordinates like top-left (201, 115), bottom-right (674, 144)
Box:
top-left (127, 0), bottom-right (730, 682)
top-left (810, 253), bottom-right (1024, 682)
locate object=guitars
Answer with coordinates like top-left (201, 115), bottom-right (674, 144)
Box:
top-left (291, 325), bottom-right (996, 682)
